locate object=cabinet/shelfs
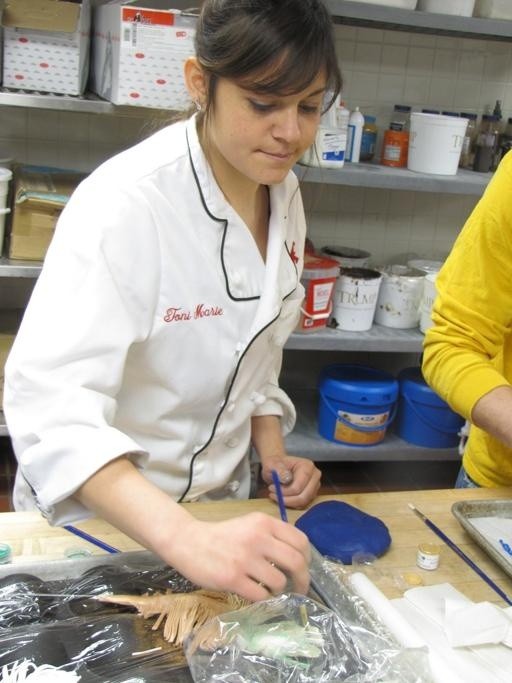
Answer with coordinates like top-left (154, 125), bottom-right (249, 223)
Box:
top-left (243, 2), bottom-right (509, 460)
top-left (3, 87), bottom-right (214, 305)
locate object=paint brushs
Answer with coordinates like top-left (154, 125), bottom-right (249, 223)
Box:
top-left (270, 468), bottom-right (309, 630)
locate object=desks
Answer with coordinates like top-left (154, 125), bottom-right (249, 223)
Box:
top-left (2, 487), bottom-right (511, 678)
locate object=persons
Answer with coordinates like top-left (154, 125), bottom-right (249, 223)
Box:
top-left (0, 0), bottom-right (350, 610)
top-left (415, 143), bottom-right (511, 495)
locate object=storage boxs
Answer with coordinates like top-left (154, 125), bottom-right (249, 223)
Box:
top-left (18, 166), bottom-right (121, 256)
top-left (93, 6), bottom-right (217, 115)
top-left (6, 1), bottom-right (90, 89)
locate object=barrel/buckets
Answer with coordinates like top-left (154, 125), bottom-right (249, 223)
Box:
top-left (0, 166), bottom-right (14, 258)
top-left (291, 252), bottom-right (341, 332)
top-left (316, 362), bottom-right (402, 447)
top-left (407, 112), bottom-right (471, 176)
top-left (397, 367), bottom-right (463, 448)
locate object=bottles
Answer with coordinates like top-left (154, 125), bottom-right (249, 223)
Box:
top-left (346, 104), bottom-right (511, 172)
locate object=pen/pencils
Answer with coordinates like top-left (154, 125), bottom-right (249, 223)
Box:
top-left (408, 502), bottom-right (512, 606)
top-left (65, 525), bottom-right (121, 553)
